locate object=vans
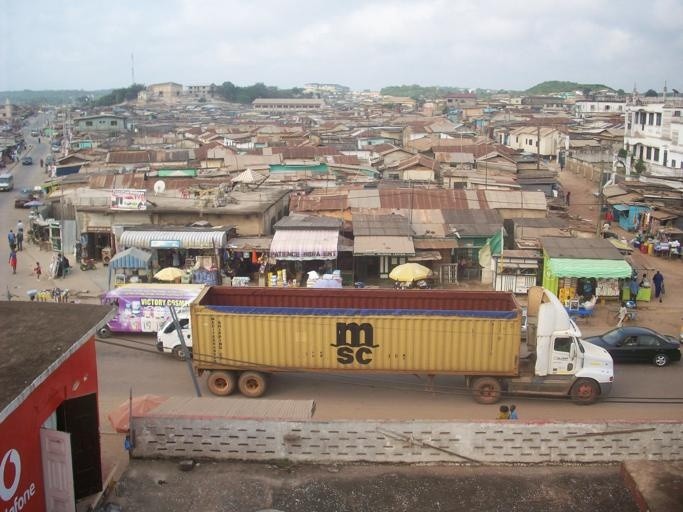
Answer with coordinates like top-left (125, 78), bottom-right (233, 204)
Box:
top-left (0, 173), bottom-right (13, 192)
top-left (95, 282), bottom-right (207, 361)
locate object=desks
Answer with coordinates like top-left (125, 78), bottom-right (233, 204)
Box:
top-left (565, 309), bottom-right (593, 328)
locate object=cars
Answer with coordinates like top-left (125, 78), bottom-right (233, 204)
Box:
top-left (579, 326), bottom-right (682, 368)
top-left (20, 155), bottom-right (32, 165)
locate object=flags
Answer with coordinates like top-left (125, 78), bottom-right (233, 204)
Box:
top-left (487, 229), bottom-right (504, 255)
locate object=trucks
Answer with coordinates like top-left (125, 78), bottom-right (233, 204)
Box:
top-left (186, 277), bottom-right (616, 404)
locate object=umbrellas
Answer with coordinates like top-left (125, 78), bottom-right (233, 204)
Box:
top-left (23, 200), bottom-right (43, 206)
top-left (107, 392), bottom-right (168, 434)
top-left (152, 266), bottom-right (186, 284)
top-left (388, 261), bottom-right (433, 282)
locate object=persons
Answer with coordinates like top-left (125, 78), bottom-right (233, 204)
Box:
top-left (624, 337), bottom-right (637, 346)
top-left (137, 194), bottom-right (146, 210)
top-left (618, 269), bottom-right (664, 304)
top-left (75, 239), bottom-right (83, 263)
top-left (509, 405), bottom-right (518, 419)
top-left (612, 301), bottom-right (627, 327)
top-left (606, 211), bottom-right (612, 226)
top-left (393, 279), bottom-right (432, 290)
top-left (601, 220), bottom-right (609, 230)
top-left (57, 254), bottom-right (69, 277)
top-left (496, 404), bottom-right (510, 419)
top-left (565, 280), bottom-right (591, 319)
top-left (6, 219), bottom-right (24, 274)
top-left (619, 236), bottom-right (628, 255)
top-left (564, 191), bottom-right (570, 206)
top-left (33, 262), bottom-right (41, 282)
